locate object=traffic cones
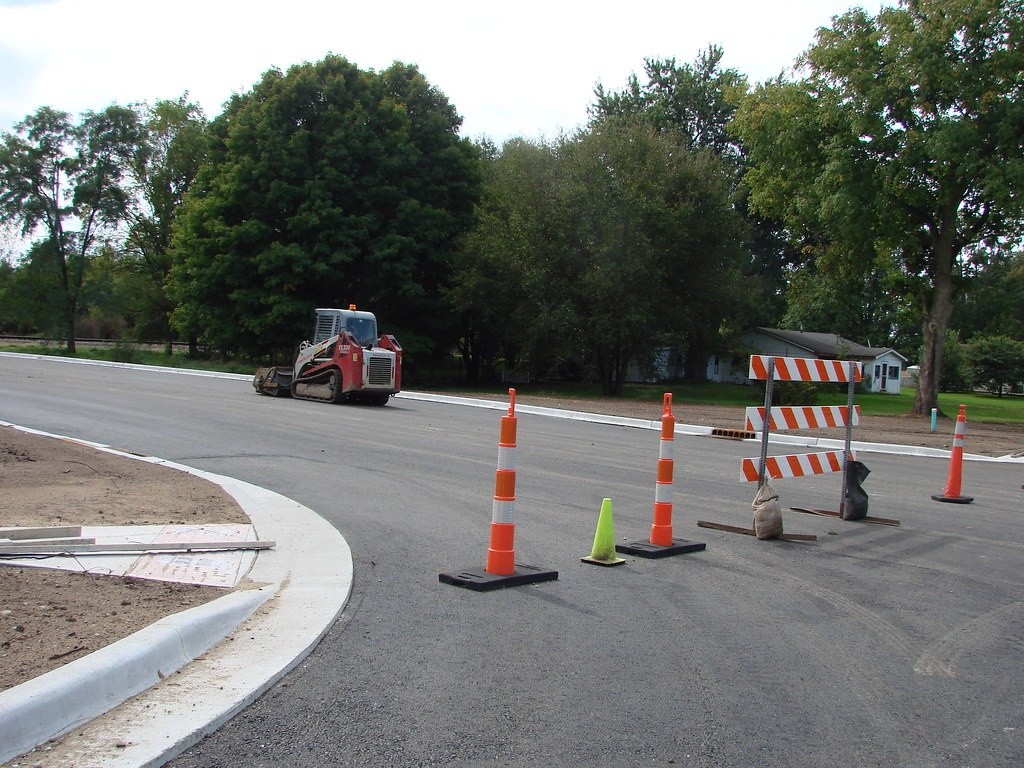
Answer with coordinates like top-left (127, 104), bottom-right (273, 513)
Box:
top-left (579, 497), bottom-right (629, 567)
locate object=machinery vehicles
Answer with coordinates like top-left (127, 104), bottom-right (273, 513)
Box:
top-left (252, 304), bottom-right (402, 406)
